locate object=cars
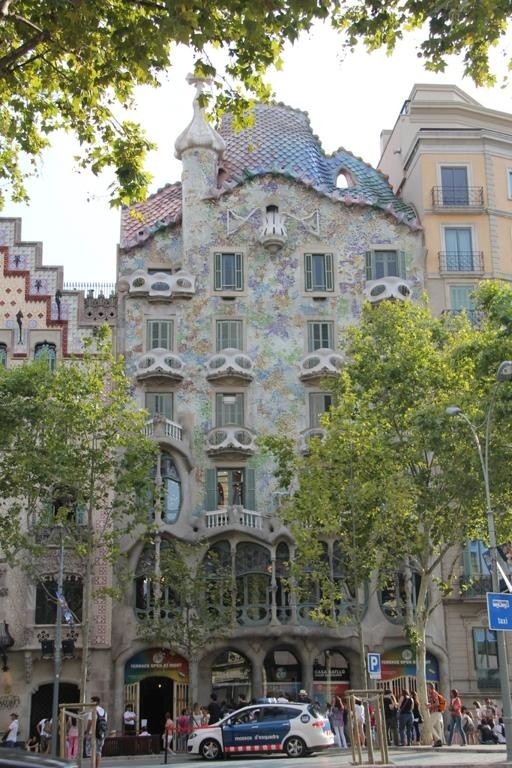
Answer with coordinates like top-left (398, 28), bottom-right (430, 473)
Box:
top-left (186, 702), bottom-right (334, 756)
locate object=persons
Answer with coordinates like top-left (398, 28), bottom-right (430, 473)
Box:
top-left (138, 727), bottom-right (151, 736)
top-left (298, 690), bottom-right (377, 750)
top-left (163, 693), bottom-right (223, 758)
top-left (5, 713), bottom-right (19, 748)
top-left (383, 689), bottom-right (421, 746)
top-left (426, 684), bottom-right (507, 747)
top-left (220, 694), bottom-right (249, 716)
top-left (37, 696), bottom-right (107, 767)
top-left (123, 704), bottom-right (137, 736)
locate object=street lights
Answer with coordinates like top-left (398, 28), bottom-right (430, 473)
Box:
top-left (445, 361), bottom-right (512, 764)
top-left (50, 522), bottom-right (70, 754)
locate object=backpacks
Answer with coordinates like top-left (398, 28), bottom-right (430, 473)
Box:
top-left (433, 692), bottom-right (445, 712)
top-left (95, 710), bottom-right (107, 741)
